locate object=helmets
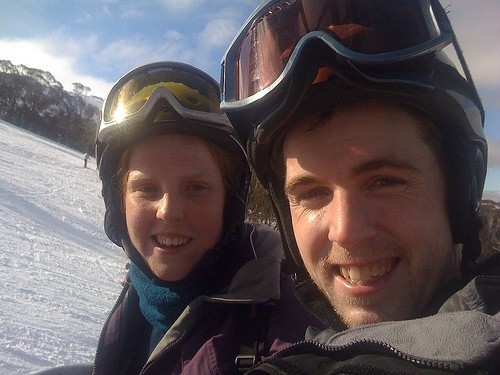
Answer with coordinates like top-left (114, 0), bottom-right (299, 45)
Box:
top-left (89, 60), bottom-right (248, 252)
top-left (217, 0), bottom-right (489, 259)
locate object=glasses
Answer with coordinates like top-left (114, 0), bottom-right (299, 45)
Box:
top-left (97, 61), bottom-right (240, 137)
top-left (220, 0), bottom-right (485, 146)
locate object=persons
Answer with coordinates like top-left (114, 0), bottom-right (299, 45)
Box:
top-left (89, 59), bottom-right (327, 375)
top-left (220, 0), bottom-right (500, 375)
top-left (83, 151), bottom-right (89, 167)
